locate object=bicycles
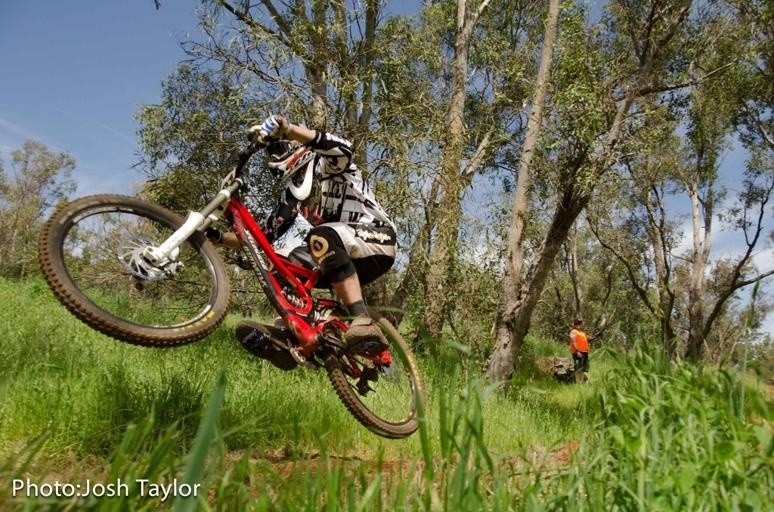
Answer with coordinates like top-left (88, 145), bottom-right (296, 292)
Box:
top-left (37, 129), bottom-right (425, 436)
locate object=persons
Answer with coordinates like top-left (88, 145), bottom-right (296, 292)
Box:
top-left (568, 319), bottom-right (589, 384)
top-left (202, 113), bottom-right (400, 372)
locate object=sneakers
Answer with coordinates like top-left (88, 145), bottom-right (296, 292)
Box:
top-left (345, 322), bottom-right (390, 355)
top-left (235, 320), bottom-right (298, 370)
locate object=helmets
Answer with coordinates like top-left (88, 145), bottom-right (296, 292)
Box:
top-left (264, 121), bottom-right (317, 183)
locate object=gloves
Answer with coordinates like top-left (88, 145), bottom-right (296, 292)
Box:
top-left (261, 115), bottom-right (294, 139)
